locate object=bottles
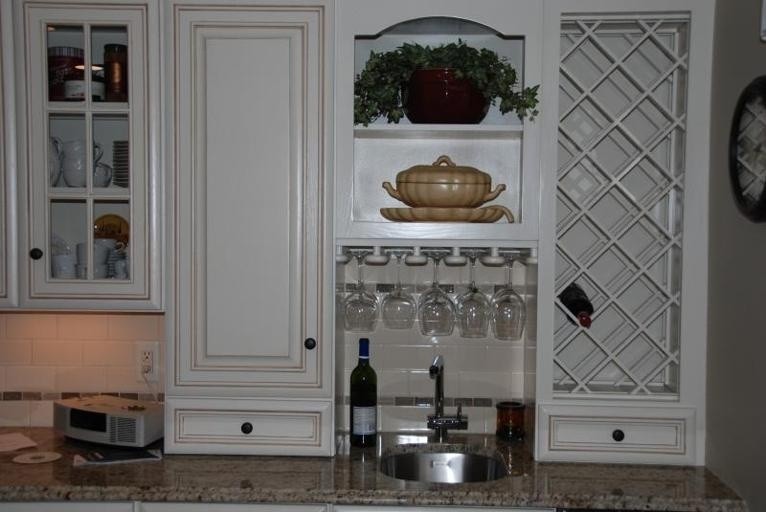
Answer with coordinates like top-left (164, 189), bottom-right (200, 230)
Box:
top-left (103, 44), bottom-right (128, 101)
top-left (349, 336), bottom-right (379, 447)
top-left (558, 283), bottom-right (597, 327)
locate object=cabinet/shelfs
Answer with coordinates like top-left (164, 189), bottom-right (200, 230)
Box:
top-left (526, 1), bottom-right (716, 470)
top-left (336, 13), bottom-right (540, 241)
top-left (16, 0), bottom-right (159, 311)
top-left (162, 1), bottom-right (343, 458)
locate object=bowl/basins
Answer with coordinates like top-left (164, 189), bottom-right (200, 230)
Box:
top-left (51, 243), bottom-right (106, 280)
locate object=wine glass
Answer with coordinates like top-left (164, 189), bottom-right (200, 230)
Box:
top-left (341, 247), bottom-right (529, 344)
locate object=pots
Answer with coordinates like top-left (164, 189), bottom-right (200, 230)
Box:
top-left (380, 155), bottom-right (506, 206)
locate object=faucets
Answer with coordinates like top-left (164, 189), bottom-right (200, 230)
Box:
top-left (428, 356), bottom-right (468, 434)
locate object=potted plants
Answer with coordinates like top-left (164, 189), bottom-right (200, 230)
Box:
top-left (354, 35), bottom-right (538, 132)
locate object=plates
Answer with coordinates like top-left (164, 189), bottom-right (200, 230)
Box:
top-left (111, 139), bottom-right (130, 187)
top-left (108, 253), bottom-right (123, 276)
top-left (377, 206), bottom-right (503, 223)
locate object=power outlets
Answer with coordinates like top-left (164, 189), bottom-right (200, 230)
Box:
top-left (134, 340), bottom-right (161, 383)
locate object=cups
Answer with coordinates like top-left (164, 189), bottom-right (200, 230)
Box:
top-left (95, 237), bottom-right (124, 252)
top-left (48, 137), bottom-right (111, 188)
top-left (495, 400), bottom-right (526, 444)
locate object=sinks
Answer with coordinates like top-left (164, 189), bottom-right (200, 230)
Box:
top-left (380, 443), bottom-right (511, 483)
